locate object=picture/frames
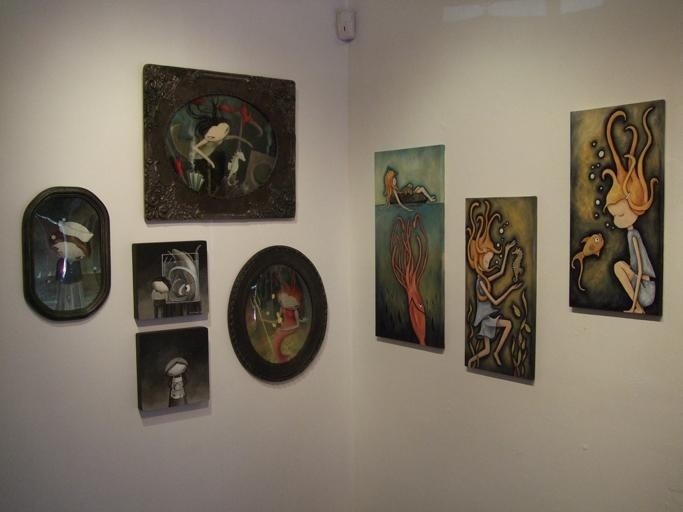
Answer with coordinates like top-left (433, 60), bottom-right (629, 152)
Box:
top-left (20, 185), bottom-right (111, 320)
top-left (143, 62), bottom-right (296, 221)
top-left (227, 244), bottom-right (328, 383)
